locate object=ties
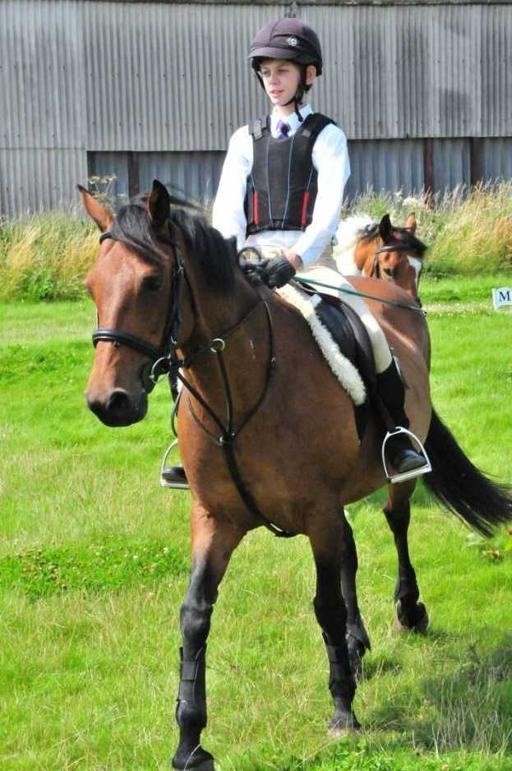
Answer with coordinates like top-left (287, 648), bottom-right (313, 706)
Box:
top-left (277, 124), bottom-right (291, 140)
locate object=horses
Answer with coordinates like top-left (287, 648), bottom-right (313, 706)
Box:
top-left (332, 211), bottom-right (428, 310)
top-left (77, 179), bottom-right (512, 771)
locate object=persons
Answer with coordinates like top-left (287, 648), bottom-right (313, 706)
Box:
top-left (160, 18), bottom-right (425, 484)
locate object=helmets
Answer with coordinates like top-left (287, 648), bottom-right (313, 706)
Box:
top-left (247, 18), bottom-right (322, 77)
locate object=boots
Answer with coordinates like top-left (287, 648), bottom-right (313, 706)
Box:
top-left (372, 357), bottom-right (425, 473)
top-left (161, 467), bottom-right (189, 484)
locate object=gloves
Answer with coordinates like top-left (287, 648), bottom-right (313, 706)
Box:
top-left (238, 254), bottom-right (297, 290)
